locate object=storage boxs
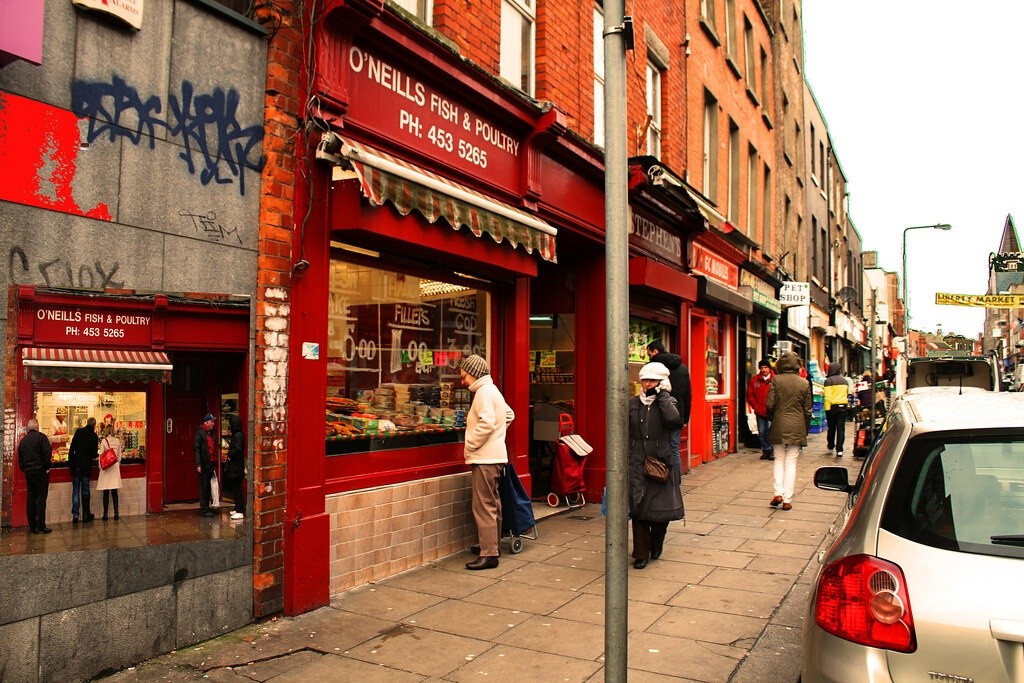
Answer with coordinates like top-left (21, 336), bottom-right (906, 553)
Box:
top-left (808, 394), bottom-right (827, 433)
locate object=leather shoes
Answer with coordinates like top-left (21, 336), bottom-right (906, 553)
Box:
top-left (651, 546), bottom-right (662, 560)
top-left (633, 559), bottom-right (647, 568)
top-left (470, 544), bottom-right (502, 557)
top-left (465, 557), bottom-right (499, 570)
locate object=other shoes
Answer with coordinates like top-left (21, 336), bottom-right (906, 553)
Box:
top-left (73, 518), bottom-right (78, 525)
top-left (114, 515), bottom-right (119, 520)
top-left (200, 511), bottom-right (215, 518)
top-left (770, 495), bottom-right (784, 506)
top-left (759, 451), bottom-right (775, 460)
top-left (837, 451), bottom-right (843, 456)
top-left (229, 510), bottom-right (244, 519)
top-left (30, 525), bottom-right (52, 534)
top-left (782, 503), bottom-right (793, 511)
top-left (83, 513), bottom-right (95, 523)
top-left (101, 516), bottom-right (108, 521)
top-left (827, 444), bottom-right (834, 452)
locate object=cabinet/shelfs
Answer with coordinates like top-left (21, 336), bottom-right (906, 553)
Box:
top-left (528, 349), bottom-right (575, 408)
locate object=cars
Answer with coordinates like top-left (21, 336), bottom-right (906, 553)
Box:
top-left (904, 387), bottom-right (986, 393)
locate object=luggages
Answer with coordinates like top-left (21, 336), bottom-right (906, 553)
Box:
top-left (500, 462), bottom-right (537, 555)
top-left (546, 413), bottom-right (594, 509)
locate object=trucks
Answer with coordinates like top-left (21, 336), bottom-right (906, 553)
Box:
top-left (906, 349), bottom-right (1002, 391)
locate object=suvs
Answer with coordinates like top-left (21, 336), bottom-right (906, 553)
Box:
top-left (801, 394), bottom-right (1024, 683)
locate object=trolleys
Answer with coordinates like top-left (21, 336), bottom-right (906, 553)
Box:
top-left (500, 462), bottom-right (538, 554)
top-left (548, 413), bottom-right (594, 508)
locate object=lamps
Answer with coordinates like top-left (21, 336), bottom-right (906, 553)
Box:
top-left (529, 314), bottom-right (559, 329)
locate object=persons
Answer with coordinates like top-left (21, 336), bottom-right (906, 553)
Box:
top-left (845, 366), bottom-right (894, 406)
top-left (824, 362), bottom-right (849, 457)
top-left (68, 417), bottom-right (99, 524)
top-left (747, 359), bottom-right (776, 460)
top-left (96, 423), bottom-right (122, 522)
top-left (766, 351), bottom-right (813, 510)
top-left (227, 416), bottom-right (244, 520)
top-left (48, 406), bottom-right (70, 452)
top-left (461, 353), bottom-right (515, 570)
top-left (194, 413), bottom-right (218, 518)
top-left (647, 340), bottom-right (692, 485)
top-left (17, 418), bottom-right (53, 536)
top-left (628, 361), bottom-right (685, 570)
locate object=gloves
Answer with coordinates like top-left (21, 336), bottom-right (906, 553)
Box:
top-left (655, 378), bottom-right (672, 393)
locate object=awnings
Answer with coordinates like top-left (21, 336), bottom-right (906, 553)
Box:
top-left (22, 347), bottom-right (174, 370)
top-left (314, 129), bottom-right (558, 266)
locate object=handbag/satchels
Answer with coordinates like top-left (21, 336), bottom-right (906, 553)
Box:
top-left (746, 405), bottom-right (759, 434)
top-left (644, 456), bottom-right (670, 481)
top-left (600, 487), bottom-right (607, 519)
top-left (209, 471), bottom-right (220, 508)
top-left (99, 438), bottom-right (118, 471)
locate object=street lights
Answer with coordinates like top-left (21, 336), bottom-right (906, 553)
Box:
top-left (902, 223), bottom-right (952, 356)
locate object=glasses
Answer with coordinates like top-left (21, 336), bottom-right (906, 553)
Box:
top-left (462, 372), bottom-right (469, 381)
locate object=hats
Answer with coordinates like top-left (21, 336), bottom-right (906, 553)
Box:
top-left (202, 414), bottom-right (216, 424)
top-left (461, 354), bottom-right (489, 378)
top-left (758, 359), bottom-right (771, 369)
top-left (55, 408), bottom-right (67, 416)
top-left (638, 362), bottom-right (670, 379)
